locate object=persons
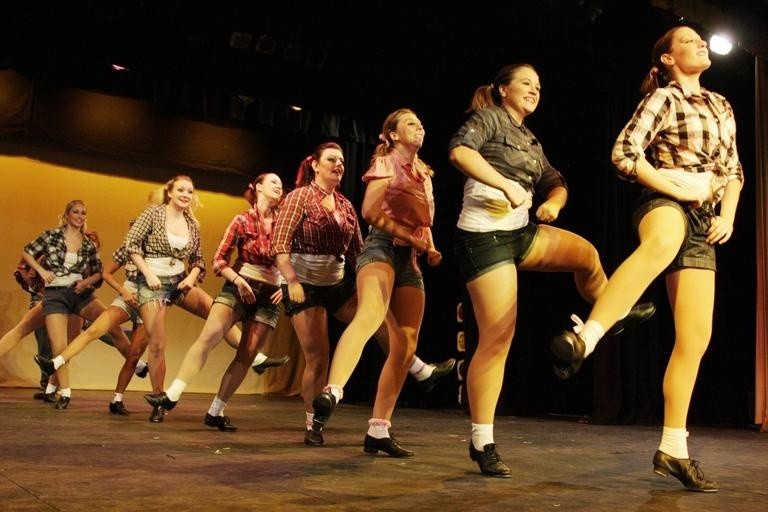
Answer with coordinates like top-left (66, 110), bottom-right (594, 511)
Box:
top-left (273, 139), bottom-right (456, 442)
top-left (145, 175), bottom-right (284, 434)
top-left (548, 24), bottom-right (745, 492)
top-left (31, 218), bottom-right (149, 416)
top-left (447, 60), bottom-right (659, 476)
top-left (309, 109), bottom-right (444, 458)
top-left (125, 174), bottom-right (288, 423)
top-left (1, 199), bottom-right (149, 409)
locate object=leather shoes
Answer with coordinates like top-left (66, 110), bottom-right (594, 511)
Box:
top-left (44, 392), bottom-right (57, 402)
top-left (550, 329), bottom-right (586, 379)
top-left (417, 358), bottom-right (456, 393)
top-left (136, 362), bottom-right (149, 378)
top-left (33, 354), bottom-right (57, 376)
top-left (312, 391), bottom-right (335, 431)
top-left (34, 391), bottom-right (44, 400)
top-left (469, 439), bottom-right (511, 477)
top-left (653, 450), bottom-right (720, 492)
top-left (607, 302), bottom-right (657, 336)
top-left (252, 356), bottom-right (290, 376)
top-left (204, 412), bottom-right (238, 431)
top-left (304, 430), bottom-right (323, 446)
top-left (109, 400), bottom-right (128, 416)
top-left (144, 392), bottom-right (178, 411)
top-left (363, 434), bottom-right (414, 458)
top-left (149, 405), bottom-right (165, 423)
top-left (55, 396), bottom-right (70, 410)
